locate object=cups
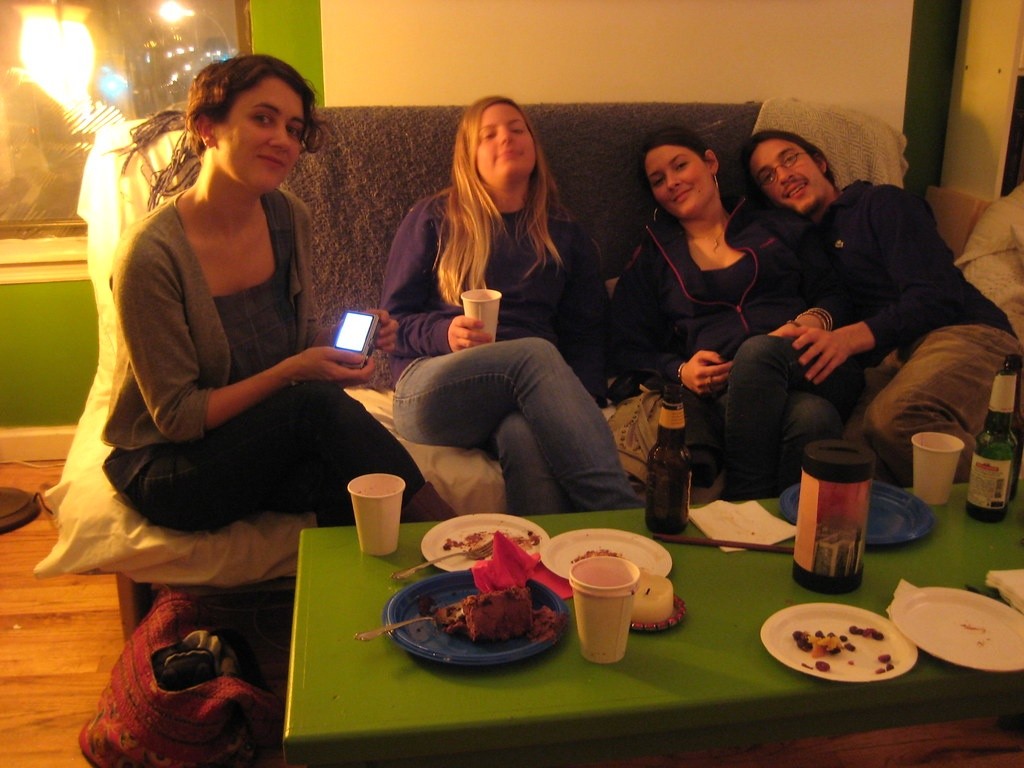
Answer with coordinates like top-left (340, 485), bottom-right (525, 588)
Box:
top-left (568, 555), bottom-right (640, 664)
top-left (347, 474), bottom-right (405, 556)
top-left (461, 289), bottom-right (502, 342)
top-left (911, 432), bottom-right (965, 505)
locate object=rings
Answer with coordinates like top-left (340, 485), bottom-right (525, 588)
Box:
top-left (707, 377), bottom-right (714, 390)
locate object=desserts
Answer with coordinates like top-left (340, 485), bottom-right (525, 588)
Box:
top-left (444, 586), bottom-right (537, 643)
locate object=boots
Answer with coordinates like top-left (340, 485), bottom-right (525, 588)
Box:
top-left (400, 481), bottom-right (457, 521)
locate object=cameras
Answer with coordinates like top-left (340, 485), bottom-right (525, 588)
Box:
top-left (333, 309), bottom-right (380, 370)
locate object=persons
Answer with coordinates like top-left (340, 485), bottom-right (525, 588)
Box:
top-left (741, 130), bottom-right (1020, 486)
top-left (380, 97), bottom-right (638, 520)
top-left (612, 126), bottom-right (867, 502)
top-left (99, 55), bottom-right (462, 533)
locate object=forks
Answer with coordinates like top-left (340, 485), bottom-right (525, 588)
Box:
top-left (390, 540), bottom-right (493, 579)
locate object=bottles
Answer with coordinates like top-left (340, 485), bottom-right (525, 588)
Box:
top-left (792, 439), bottom-right (877, 594)
top-left (964, 369), bottom-right (1018, 524)
top-left (644, 383), bottom-right (692, 535)
top-left (998, 353), bottom-right (1023, 501)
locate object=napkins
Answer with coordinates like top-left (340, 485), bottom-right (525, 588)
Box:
top-left (469, 530), bottom-right (538, 592)
top-left (527, 562), bottom-right (573, 600)
top-left (885, 578), bottom-right (919, 619)
top-left (985, 568), bottom-right (1024, 613)
top-left (689, 500), bottom-right (796, 555)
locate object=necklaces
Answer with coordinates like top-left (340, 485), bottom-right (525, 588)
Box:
top-left (685, 228), bottom-right (726, 253)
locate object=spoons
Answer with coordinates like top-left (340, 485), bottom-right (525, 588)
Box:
top-left (355, 602), bottom-right (463, 640)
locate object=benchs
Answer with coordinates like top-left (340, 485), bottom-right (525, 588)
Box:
top-left (32, 100), bottom-right (925, 647)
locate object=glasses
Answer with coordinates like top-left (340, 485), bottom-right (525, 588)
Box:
top-left (757, 152), bottom-right (806, 189)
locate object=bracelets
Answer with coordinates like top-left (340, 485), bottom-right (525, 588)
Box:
top-left (793, 308), bottom-right (833, 331)
top-left (678, 361), bottom-right (685, 389)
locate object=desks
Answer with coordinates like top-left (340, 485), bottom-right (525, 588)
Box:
top-left (283, 482), bottom-right (1024, 768)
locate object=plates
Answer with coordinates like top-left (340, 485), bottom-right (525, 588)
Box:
top-left (890, 586), bottom-right (1024, 673)
top-left (421, 513), bottom-right (550, 570)
top-left (760, 602), bottom-right (918, 681)
top-left (381, 570), bottom-right (570, 666)
top-left (780, 481), bottom-right (935, 545)
top-left (539, 529), bottom-right (672, 580)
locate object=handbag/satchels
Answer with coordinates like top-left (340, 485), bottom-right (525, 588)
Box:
top-left (78, 585), bottom-right (284, 768)
